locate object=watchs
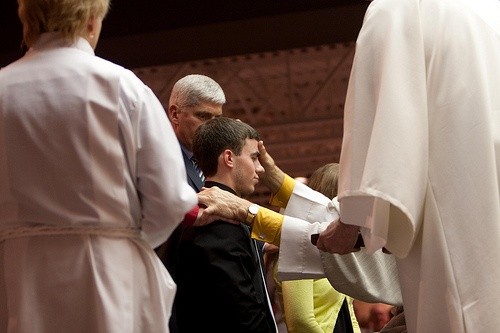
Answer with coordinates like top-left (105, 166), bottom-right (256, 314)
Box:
top-left (246, 204), bottom-right (259, 223)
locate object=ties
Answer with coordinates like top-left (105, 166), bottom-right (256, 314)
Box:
top-left (190, 156), bottom-right (206, 185)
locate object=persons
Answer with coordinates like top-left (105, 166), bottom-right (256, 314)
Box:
top-left (167, 118), bottom-right (279, 333)
top-left (317, 0), bottom-right (500, 333)
top-left (0, 0), bottom-right (199, 333)
top-left (260, 163), bottom-right (393, 333)
top-left (195, 120), bottom-right (406, 333)
top-left (169, 74), bottom-right (226, 192)
